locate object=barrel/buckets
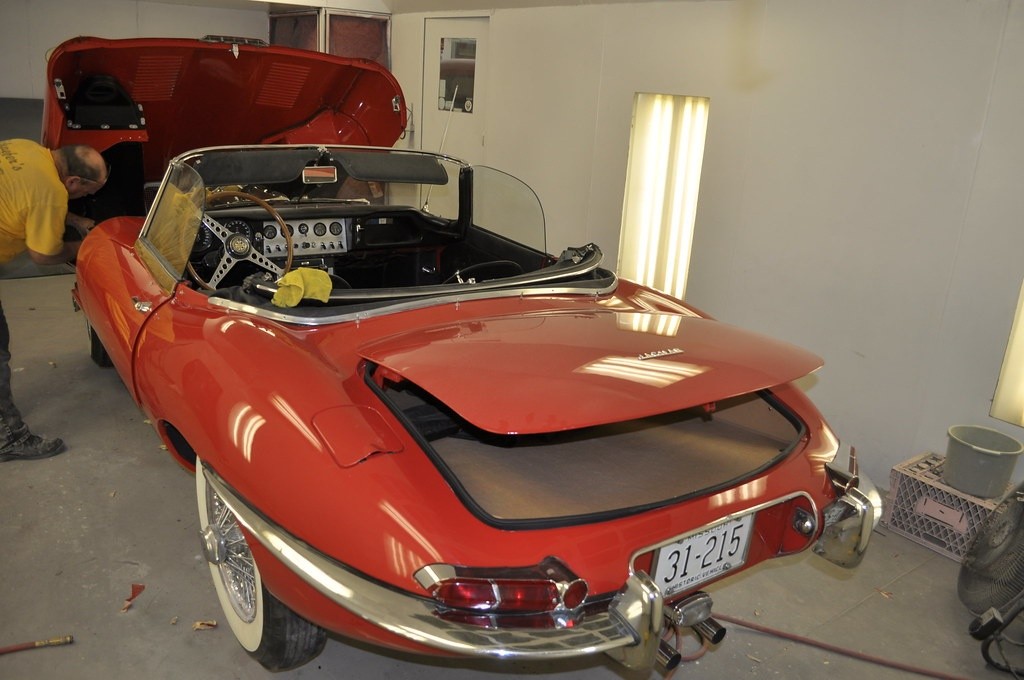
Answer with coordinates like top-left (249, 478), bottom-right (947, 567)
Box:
top-left (944, 423), bottom-right (1023, 500)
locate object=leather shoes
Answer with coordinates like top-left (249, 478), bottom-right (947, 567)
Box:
top-left (1, 433), bottom-right (63, 461)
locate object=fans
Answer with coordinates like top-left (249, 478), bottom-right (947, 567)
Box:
top-left (956, 481), bottom-right (1024, 680)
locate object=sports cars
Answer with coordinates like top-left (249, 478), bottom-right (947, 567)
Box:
top-left (68, 144), bottom-right (885, 680)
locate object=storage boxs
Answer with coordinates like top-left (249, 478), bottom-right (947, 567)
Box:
top-left (881, 452), bottom-right (1019, 564)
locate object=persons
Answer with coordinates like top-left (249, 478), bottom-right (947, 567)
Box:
top-left (0, 135), bottom-right (109, 461)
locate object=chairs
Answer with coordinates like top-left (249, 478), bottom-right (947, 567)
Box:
top-left (440, 260), bottom-right (525, 285)
top-left (281, 268), bottom-right (353, 290)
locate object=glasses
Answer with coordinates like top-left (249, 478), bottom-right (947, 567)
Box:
top-left (75, 165), bottom-right (113, 185)
